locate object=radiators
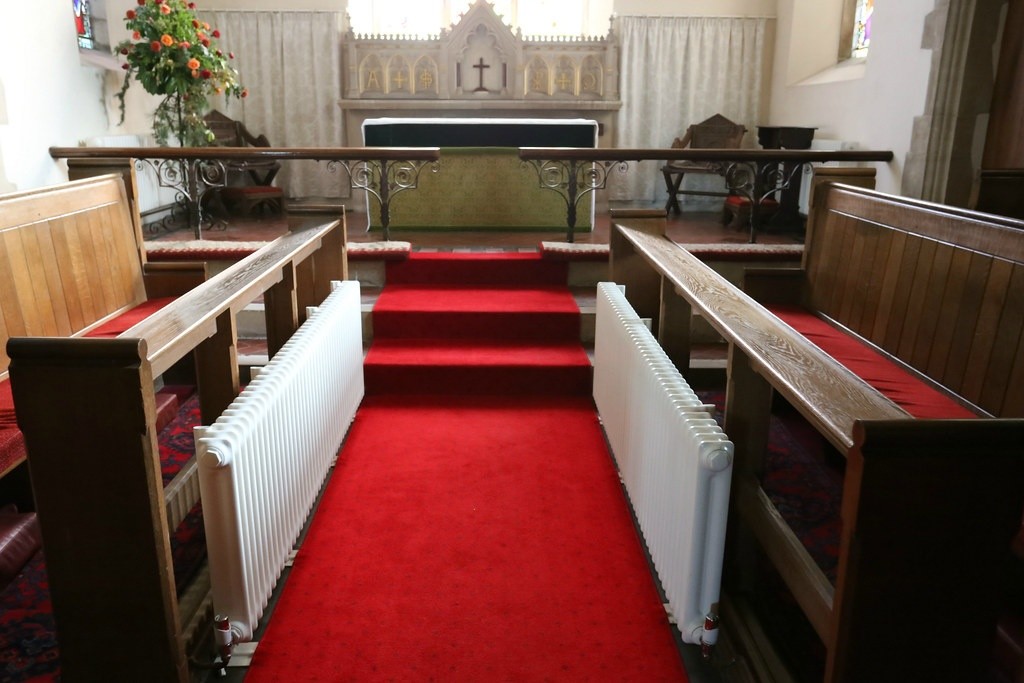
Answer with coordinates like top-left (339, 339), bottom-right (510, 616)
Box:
top-left (193, 280), bottom-right (365, 642)
top-left (592, 282), bottom-right (734, 645)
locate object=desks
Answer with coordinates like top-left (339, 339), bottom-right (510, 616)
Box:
top-left (546, 208), bottom-right (1024, 683)
top-left (6, 204), bottom-right (349, 683)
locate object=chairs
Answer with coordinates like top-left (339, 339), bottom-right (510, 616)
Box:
top-left (192, 109), bottom-right (287, 222)
top-left (660, 113), bottom-right (748, 216)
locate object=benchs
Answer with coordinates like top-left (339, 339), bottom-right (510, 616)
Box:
top-left (739, 163), bottom-right (1024, 683)
top-left (0, 172), bottom-right (210, 589)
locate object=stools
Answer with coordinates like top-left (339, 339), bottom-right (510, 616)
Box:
top-left (719, 195), bottom-right (779, 233)
top-left (222, 185), bottom-right (284, 222)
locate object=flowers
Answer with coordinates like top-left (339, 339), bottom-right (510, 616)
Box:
top-left (112, 0), bottom-right (249, 147)
